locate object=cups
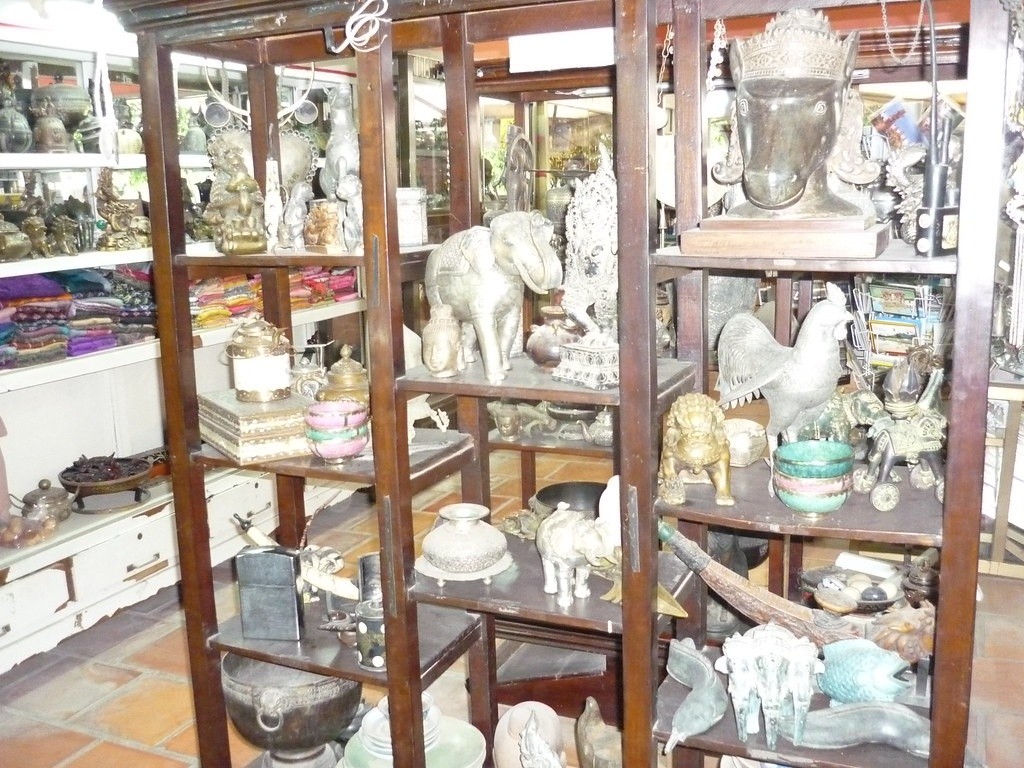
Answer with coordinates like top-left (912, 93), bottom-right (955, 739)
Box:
top-left (393, 187), bottom-right (430, 247)
top-left (354, 586), bottom-right (388, 673)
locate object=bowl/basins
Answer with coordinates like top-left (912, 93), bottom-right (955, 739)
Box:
top-left (378, 692), bottom-right (433, 723)
top-left (772, 440), bottom-right (855, 519)
top-left (837, 580), bottom-right (905, 616)
top-left (303, 401), bottom-right (370, 466)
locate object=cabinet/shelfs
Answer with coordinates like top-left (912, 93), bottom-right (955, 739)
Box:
top-left (0, 27), bottom-right (374, 675)
top-left (100, 0), bottom-right (1011, 768)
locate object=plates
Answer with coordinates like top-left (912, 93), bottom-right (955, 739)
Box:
top-left (335, 709), bottom-right (486, 768)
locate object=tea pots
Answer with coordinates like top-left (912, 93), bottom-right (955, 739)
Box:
top-left (291, 342), bottom-right (370, 409)
top-left (226, 314), bottom-right (293, 402)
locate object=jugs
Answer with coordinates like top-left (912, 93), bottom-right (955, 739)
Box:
top-left (900, 557), bottom-right (939, 608)
